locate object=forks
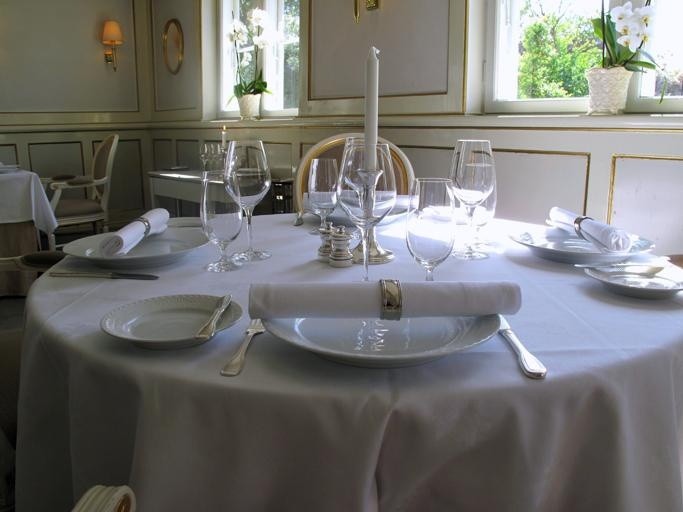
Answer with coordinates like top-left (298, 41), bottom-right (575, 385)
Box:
top-left (219, 319), bottom-right (265, 376)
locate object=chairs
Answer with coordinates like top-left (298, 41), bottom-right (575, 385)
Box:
top-left (40, 134), bottom-right (119, 250)
top-left (293, 133), bottom-right (415, 213)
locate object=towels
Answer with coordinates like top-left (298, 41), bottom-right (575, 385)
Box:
top-left (546, 206), bottom-right (632, 254)
top-left (99, 207), bottom-right (170, 256)
top-left (247, 281), bottom-right (523, 316)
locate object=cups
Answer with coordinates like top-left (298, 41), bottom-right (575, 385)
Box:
top-left (448, 139), bottom-right (497, 262)
top-left (406, 177), bottom-right (456, 281)
top-left (226, 141), bottom-right (272, 264)
top-left (215, 144), bottom-right (222, 171)
top-left (336, 137), bottom-right (398, 281)
top-left (199, 170), bottom-right (243, 273)
top-left (198, 142), bottom-right (209, 171)
top-left (308, 157), bottom-right (338, 227)
top-left (209, 142), bottom-right (215, 170)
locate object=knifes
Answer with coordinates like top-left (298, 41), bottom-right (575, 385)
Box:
top-left (48, 271), bottom-right (160, 280)
top-left (293, 211), bottom-right (303, 227)
top-left (497, 315), bottom-right (547, 379)
top-left (572, 263), bottom-right (655, 268)
top-left (194, 294), bottom-right (233, 339)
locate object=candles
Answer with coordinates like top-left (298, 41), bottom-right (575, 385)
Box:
top-left (364, 47), bottom-right (379, 171)
top-left (221, 131), bottom-right (228, 148)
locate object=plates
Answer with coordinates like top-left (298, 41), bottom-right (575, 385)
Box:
top-left (585, 261), bottom-right (683, 298)
top-left (260, 315), bottom-right (502, 368)
top-left (329, 194), bottom-right (407, 227)
top-left (0, 163), bottom-right (21, 172)
top-left (102, 294), bottom-right (244, 351)
top-left (63, 226), bottom-right (209, 270)
top-left (509, 225), bottom-right (656, 264)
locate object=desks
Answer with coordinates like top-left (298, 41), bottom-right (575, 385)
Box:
top-left (148, 170), bottom-right (261, 209)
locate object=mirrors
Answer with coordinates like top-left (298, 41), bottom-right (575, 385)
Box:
top-left (163, 18), bottom-right (184, 74)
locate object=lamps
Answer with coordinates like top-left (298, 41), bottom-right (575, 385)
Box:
top-left (102, 20), bottom-right (126, 72)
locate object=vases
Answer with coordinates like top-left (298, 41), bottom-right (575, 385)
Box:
top-left (585, 71), bottom-right (634, 116)
top-left (236, 92), bottom-right (262, 121)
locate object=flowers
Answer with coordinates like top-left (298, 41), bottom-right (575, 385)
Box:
top-left (589, 0), bottom-right (657, 73)
top-left (228, 7), bottom-right (280, 97)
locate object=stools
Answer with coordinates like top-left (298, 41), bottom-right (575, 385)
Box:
top-left (270, 180), bottom-right (293, 213)
